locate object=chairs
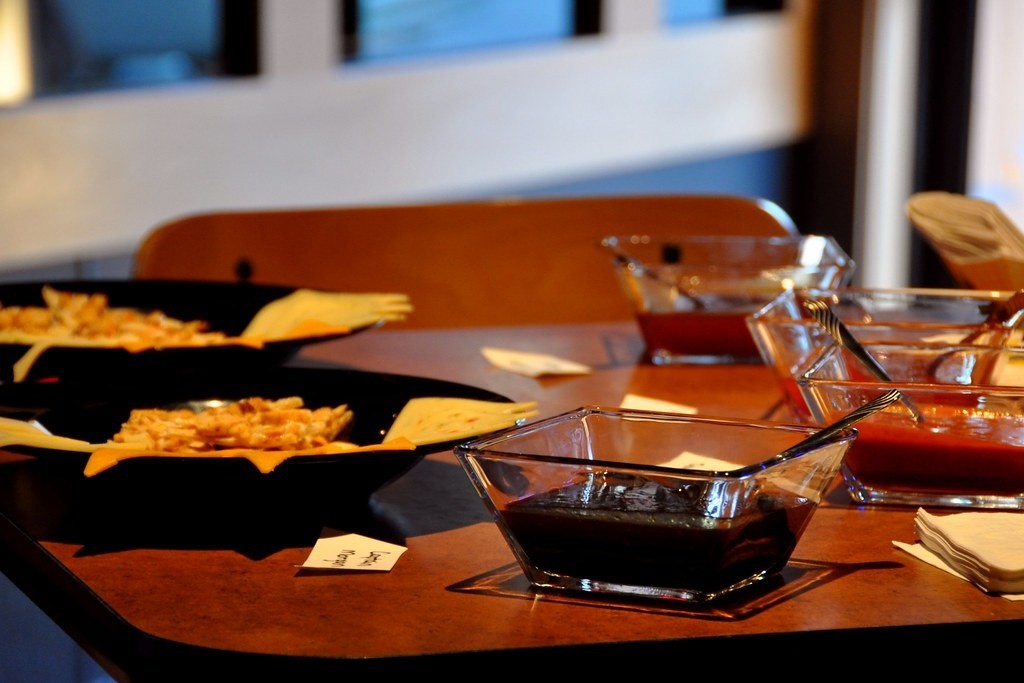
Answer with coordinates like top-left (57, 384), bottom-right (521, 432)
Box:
top-left (129, 189), bottom-right (810, 326)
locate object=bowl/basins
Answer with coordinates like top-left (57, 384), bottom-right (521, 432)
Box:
top-left (1, 277), bottom-right (382, 363)
top-left (794, 341), bottom-right (1024, 511)
top-left (600, 235), bottom-right (856, 367)
top-left (1, 366), bottom-right (515, 514)
top-left (746, 286), bottom-right (1024, 426)
top-left (452, 403), bottom-right (858, 604)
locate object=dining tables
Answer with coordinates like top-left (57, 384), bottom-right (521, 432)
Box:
top-left (0, 324), bottom-right (1024, 683)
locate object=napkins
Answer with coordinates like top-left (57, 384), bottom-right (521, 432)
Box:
top-left (891, 506), bottom-right (1024, 602)
